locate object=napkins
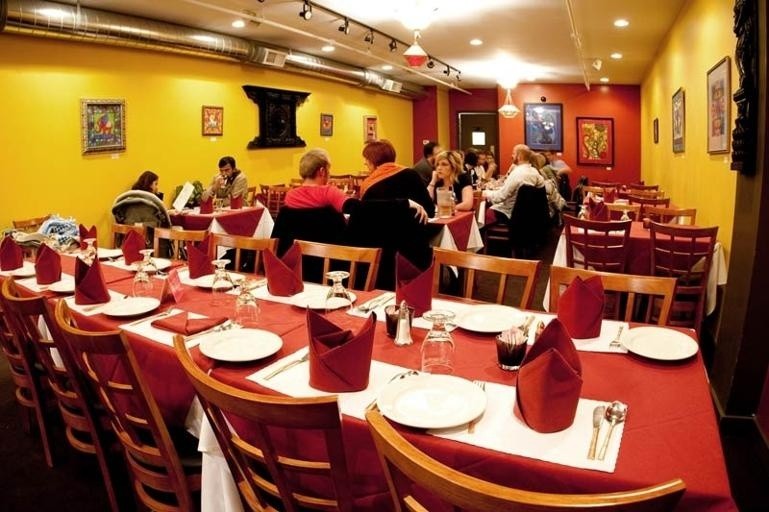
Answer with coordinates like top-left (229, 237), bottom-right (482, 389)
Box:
top-left (186, 233), bottom-right (216, 279)
top-left (394, 252), bottom-right (436, 317)
top-left (261, 241), bottom-right (304, 297)
top-left (1, 235), bottom-right (24, 272)
top-left (121, 229), bottom-right (146, 266)
top-left (512, 318), bottom-right (585, 433)
top-left (152, 311), bottom-right (229, 336)
top-left (73, 255), bottom-right (112, 305)
top-left (558, 276), bottom-right (603, 339)
top-left (34, 242), bottom-right (63, 285)
top-left (304, 303), bottom-right (378, 393)
top-left (78, 223), bottom-right (98, 251)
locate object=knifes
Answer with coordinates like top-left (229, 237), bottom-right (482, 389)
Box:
top-left (585, 403), bottom-right (607, 458)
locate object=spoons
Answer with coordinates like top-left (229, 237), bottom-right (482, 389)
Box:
top-left (357, 292), bottom-right (395, 312)
top-left (366, 370), bottom-right (418, 413)
top-left (599, 397), bottom-right (628, 461)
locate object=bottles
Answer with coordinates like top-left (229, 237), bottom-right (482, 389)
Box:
top-left (392, 299), bottom-right (414, 345)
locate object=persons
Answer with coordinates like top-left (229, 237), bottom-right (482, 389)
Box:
top-left (202, 156), bottom-right (248, 205)
top-left (284, 148), bottom-right (429, 225)
top-left (346, 139), bottom-right (435, 289)
top-left (414, 143), bottom-right (571, 229)
top-left (132, 171), bottom-right (183, 217)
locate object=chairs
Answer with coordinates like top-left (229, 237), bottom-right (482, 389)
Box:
top-left (561, 180), bottom-right (723, 340)
top-left (549, 265), bottom-right (678, 327)
top-left (293, 238), bottom-right (383, 292)
top-left (364, 407), bottom-right (687, 511)
top-left (153, 227), bottom-right (208, 262)
top-left (55, 298), bottom-right (202, 512)
top-left (208, 230), bottom-right (279, 277)
top-left (109, 224), bottom-right (146, 253)
top-left (172, 335), bottom-right (354, 512)
top-left (13, 217), bottom-right (50, 233)
top-left (431, 246), bottom-right (540, 311)
top-left (0, 275), bottom-right (123, 512)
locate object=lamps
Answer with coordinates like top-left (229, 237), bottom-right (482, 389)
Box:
top-left (497, 88), bottom-right (522, 120)
top-left (258, 0), bottom-right (466, 83)
top-left (403, 29), bottom-right (429, 70)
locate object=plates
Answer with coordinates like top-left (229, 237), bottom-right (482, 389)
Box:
top-left (377, 375), bottom-right (490, 430)
top-left (125, 256), bottom-right (171, 273)
top-left (194, 271), bottom-right (244, 289)
top-left (43, 273), bottom-right (78, 294)
top-left (620, 325), bottom-right (699, 365)
top-left (101, 298), bottom-right (162, 318)
top-left (0, 258), bottom-right (39, 277)
top-left (197, 327), bottom-right (284, 362)
top-left (453, 302), bottom-right (525, 334)
top-left (98, 247), bottom-right (123, 259)
top-left (290, 287), bottom-right (356, 311)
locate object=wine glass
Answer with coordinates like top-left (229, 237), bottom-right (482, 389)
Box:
top-left (231, 277), bottom-right (262, 325)
top-left (83, 238), bottom-right (97, 260)
top-left (139, 247), bottom-right (154, 263)
top-left (128, 260), bottom-right (155, 297)
top-left (209, 260), bottom-right (235, 301)
top-left (421, 310), bottom-right (458, 377)
top-left (215, 198), bottom-right (225, 214)
top-left (322, 271), bottom-right (355, 324)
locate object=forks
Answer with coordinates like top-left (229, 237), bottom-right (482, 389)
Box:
top-left (607, 324), bottom-right (625, 352)
top-left (265, 354), bottom-right (310, 381)
top-left (465, 376), bottom-right (488, 429)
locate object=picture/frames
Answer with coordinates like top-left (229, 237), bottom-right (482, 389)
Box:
top-left (574, 115), bottom-right (616, 168)
top-left (706, 56), bottom-right (732, 154)
top-left (651, 118), bottom-right (659, 145)
top-left (523, 102), bottom-right (563, 153)
top-left (671, 86), bottom-right (686, 154)
top-left (200, 104), bottom-right (224, 136)
top-left (320, 112), bottom-right (333, 137)
top-left (364, 114), bottom-right (378, 144)
top-left (80, 97), bottom-right (126, 156)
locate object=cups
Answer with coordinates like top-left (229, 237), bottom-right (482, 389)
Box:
top-left (497, 328), bottom-right (528, 372)
top-left (384, 304), bottom-right (415, 337)
top-left (620, 209), bottom-right (630, 221)
top-left (577, 205), bottom-right (589, 218)
top-left (436, 186), bottom-right (456, 218)
